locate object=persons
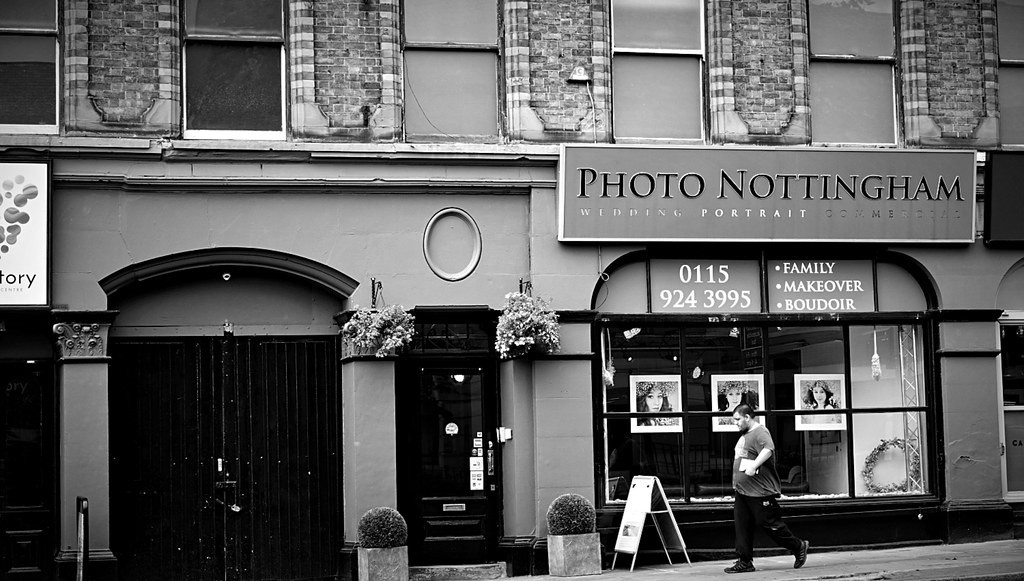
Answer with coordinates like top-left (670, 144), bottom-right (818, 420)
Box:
top-left (802, 380), bottom-right (842, 423)
top-left (722, 402), bottom-right (808, 573)
top-left (635, 380), bottom-right (677, 426)
top-left (718, 381), bottom-right (758, 426)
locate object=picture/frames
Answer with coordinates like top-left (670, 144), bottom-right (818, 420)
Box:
top-left (629, 375), bottom-right (682, 432)
top-left (792, 373), bottom-right (847, 431)
top-left (710, 374), bottom-right (766, 432)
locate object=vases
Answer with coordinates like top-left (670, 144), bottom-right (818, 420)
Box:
top-left (341, 338), bottom-right (396, 360)
top-left (508, 342), bottom-right (550, 357)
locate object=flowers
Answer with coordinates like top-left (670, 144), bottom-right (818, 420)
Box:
top-left (335, 303), bottom-right (419, 358)
top-left (494, 290), bottom-right (561, 359)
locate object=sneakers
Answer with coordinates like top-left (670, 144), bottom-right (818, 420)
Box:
top-left (794, 540), bottom-right (810, 568)
top-left (724, 558), bottom-right (755, 575)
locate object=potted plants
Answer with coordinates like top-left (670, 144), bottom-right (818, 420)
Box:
top-left (546, 493), bottom-right (602, 576)
top-left (358, 506), bottom-right (409, 581)
